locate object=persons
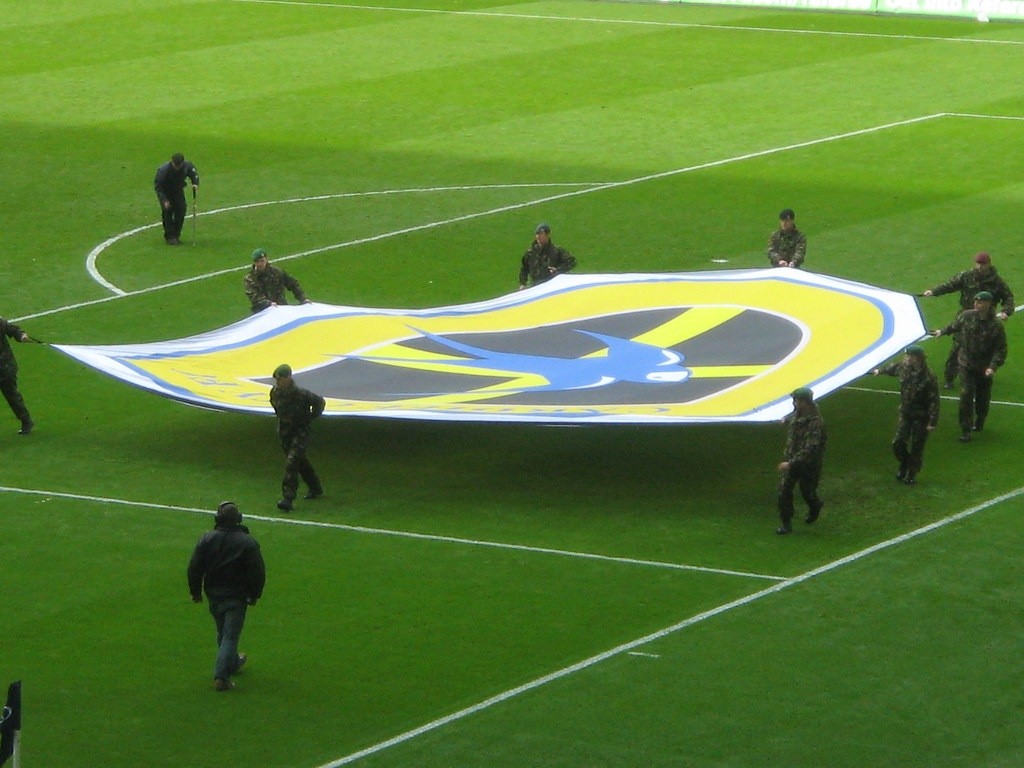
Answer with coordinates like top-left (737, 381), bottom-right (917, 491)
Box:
top-left (775, 389), bottom-right (828, 535)
top-left (270, 364), bottom-right (325, 511)
top-left (519, 225), bottom-right (577, 289)
top-left (244, 248), bottom-right (312, 315)
top-left (924, 252), bottom-right (1014, 391)
top-left (872, 345), bottom-right (940, 480)
top-left (187, 502), bottom-right (265, 689)
top-left (768, 209), bottom-right (807, 267)
top-left (0, 316), bottom-right (34, 435)
top-left (935, 291), bottom-right (1007, 443)
top-left (154, 153), bottom-right (199, 244)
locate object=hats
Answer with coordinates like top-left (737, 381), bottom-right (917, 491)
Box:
top-left (171, 153), bottom-right (184, 164)
top-left (252, 249), bottom-right (266, 262)
top-left (273, 364), bottom-right (291, 378)
top-left (905, 345), bottom-right (924, 355)
top-left (791, 388), bottom-right (813, 399)
top-left (974, 291), bottom-right (993, 301)
top-left (536, 223), bottom-right (550, 233)
top-left (976, 252), bottom-right (991, 263)
top-left (779, 209), bottom-right (794, 220)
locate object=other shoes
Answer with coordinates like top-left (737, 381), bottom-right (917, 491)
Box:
top-left (304, 485), bottom-right (323, 499)
top-left (278, 499), bottom-right (293, 512)
top-left (959, 436), bottom-right (971, 442)
top-left (972, 426), bottom-right (983, 431)
top-left (944, 382), bottom-right (953, 389)
top-left (776, 525), bottom-right (792, 534)
top-left (896, 467), bottom-right (908, 480)
top-left (905, 471), bottom-right (916, 484)
top-left (216, 678), bottom-right (235, 692)
top-left (19, 419), bottom-right (33, 435)
top-left (805, 502), bottom-right (822, 524)
top-left (232, 652), bottom-right (247, 675)
top-left (166, 238), bottom-right (181, 245)
